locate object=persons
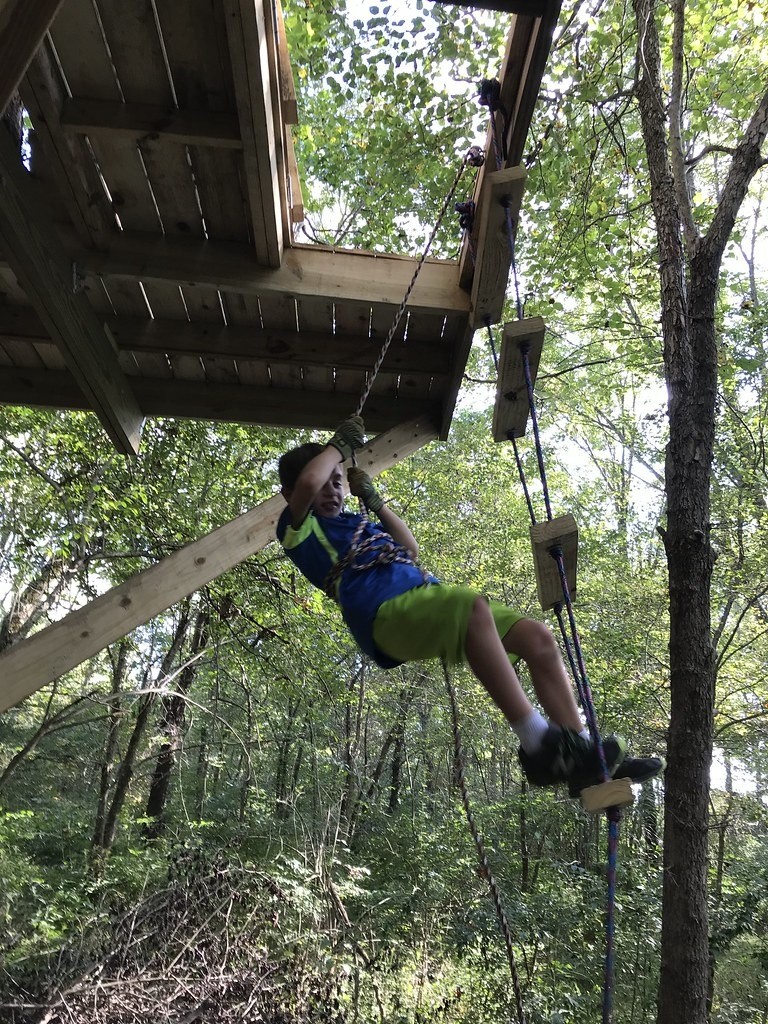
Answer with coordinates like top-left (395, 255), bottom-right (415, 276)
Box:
top-left (277, 413), bottom-right (666, 797)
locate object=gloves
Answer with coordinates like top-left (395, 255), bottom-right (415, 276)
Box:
top-left (346, 467), bottom-right (384, 512)
top-left (326, 412), bottom-right (365, 463)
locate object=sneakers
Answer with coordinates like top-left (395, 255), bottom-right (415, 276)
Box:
top-left (517, 723), bottom-right (625, 787)
top-left (568, 756), bottom-right (663, 799)
